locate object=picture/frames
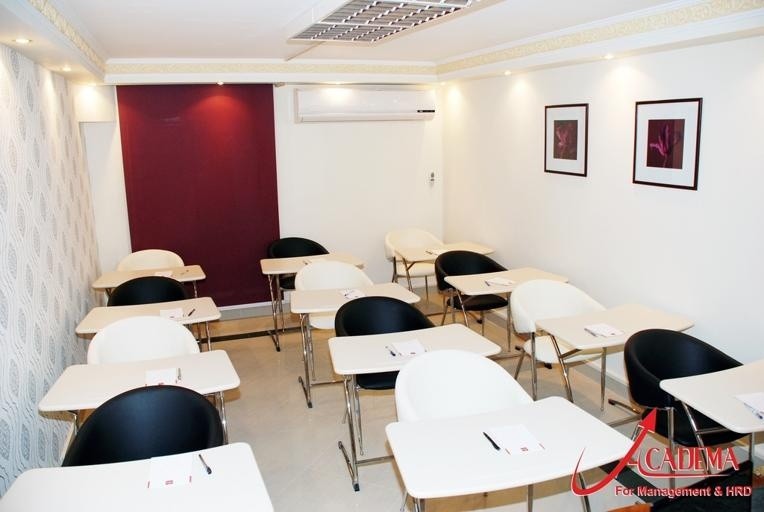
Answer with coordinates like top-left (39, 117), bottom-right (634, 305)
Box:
top-left (629, 95), bottom-right (704, 192)
top-left (541, 101), bottom-right (589, 177)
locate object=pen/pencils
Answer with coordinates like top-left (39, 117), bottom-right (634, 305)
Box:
top-left (483, 432), bottom-right (500, 451)
top-left (178, 368), bottom-right (182, 380)
top-left (485, 281), bottom-right (490, 286)
top-left (188, 309), bottom-right (195, 316)
top-left (744, 403), bottom-right (762, 419)
top-left (584, 328), bottom-right (595, 337)
top-left (199, 454), bottom-right (212, 474)
top-left (385, 346), bottom-right (396, 356)
top-left (425, 250), bottom-right (435, 256)
top-left (345, 290), bottom-right (353, 296)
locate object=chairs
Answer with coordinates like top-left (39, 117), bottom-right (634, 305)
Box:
top-left (0, 229), bottom-right (763, 512)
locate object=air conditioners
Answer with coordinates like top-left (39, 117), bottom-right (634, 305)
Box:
top-left (292, 84), bottom-right (437, 127)
